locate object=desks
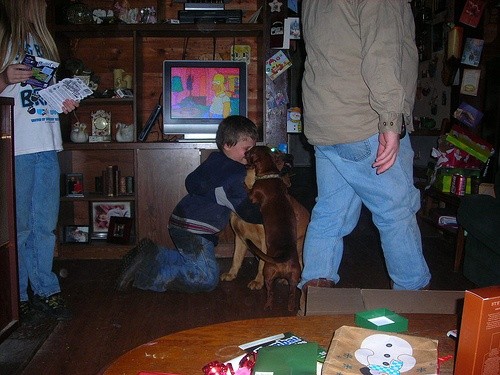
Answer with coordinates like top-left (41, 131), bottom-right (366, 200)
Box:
top-left (96, 314), bottom-right (462, 375)
top-left (409, 127), bottom-right (442, 184)
top-left (415, 182), bottom-right (466, 274)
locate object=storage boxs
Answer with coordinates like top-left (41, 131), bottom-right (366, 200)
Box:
top-left (177, 10), bottom-right (242, 24)
top-left (454, 285), bottom-right (500, 375)
top-left (306, 285), bottom-right (469, 313)
top-left (355, 308), bottom-right (407, 333)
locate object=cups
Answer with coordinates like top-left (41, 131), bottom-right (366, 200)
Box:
top-left (126, 176), bottom-right (133, 193)
top-left (95, 176), bottom-right (104, 194)
top-left (447, 27), bottom-right (463, 60)
top-left (102, 165), bottom-right (119, 197)
top-left (119, 176), bottom-right (126, 194)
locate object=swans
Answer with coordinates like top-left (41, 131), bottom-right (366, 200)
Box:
top-left (116, 123), bottom-right (133, 142)
top-left (71, 123), bottom-right (89, 143)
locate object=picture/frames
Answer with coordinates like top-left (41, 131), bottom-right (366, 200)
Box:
top-left (62, 201), bottom-right (134, 244)
top-left (64, 173), bottom-right (85, 197)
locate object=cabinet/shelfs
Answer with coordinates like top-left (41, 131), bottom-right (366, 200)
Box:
top-left (0, 96), bottom-right (21, 344)
top-left (48, 0), bottom-right (289, 261)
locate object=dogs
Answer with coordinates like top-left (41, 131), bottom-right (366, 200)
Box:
top-left (220, 150), bottom-right (311, 291)
top-left (242, 146), bottom-right (302, 315)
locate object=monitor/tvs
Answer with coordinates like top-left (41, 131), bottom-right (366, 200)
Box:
top-left (163, 59), bottom-right (248, 142)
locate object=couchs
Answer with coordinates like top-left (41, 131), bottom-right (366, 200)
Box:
top-left (456, 193), bottom-right (499, 288)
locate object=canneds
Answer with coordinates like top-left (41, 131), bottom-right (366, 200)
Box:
top-left (450, 172), bottom-right (466, 196)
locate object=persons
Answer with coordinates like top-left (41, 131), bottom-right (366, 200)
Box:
top-left (67, 176), bottom-right (83, 195)
top-left (0, 0), bottom-right (79, 318)
top-left (107, 115), bottom-right (265, 293)
top-left (297, 0), bottom-right (432, 311)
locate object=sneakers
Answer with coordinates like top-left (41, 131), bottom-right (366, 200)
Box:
top-left (114, 238), bottom-right (158, 289)
top-left (19, 293), bottom-right (73, 319)
